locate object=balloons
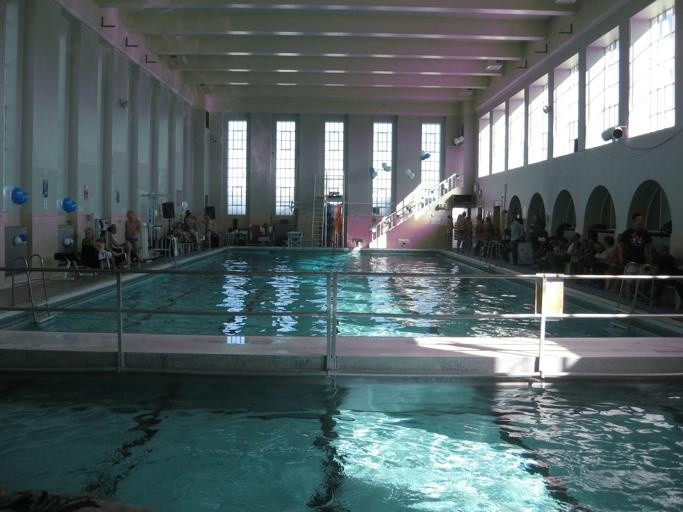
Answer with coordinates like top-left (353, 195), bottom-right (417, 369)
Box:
top-left (368, 166), bottom-right (377, 180)
top-left (452, 136), bottom-right (464, 145)
top-left (405, 169), bottom-right (415, 179)
top-left (419, 151), bottom-right (430, 160)
top-left (382, 163), bottom-right (391, 172)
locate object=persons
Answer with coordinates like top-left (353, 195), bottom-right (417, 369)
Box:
top-left (446, 211), bottom-right (683, 299)
top-left (81, 210), bottom-right (214, 271)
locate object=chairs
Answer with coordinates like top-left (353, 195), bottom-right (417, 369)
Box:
top-left (223, 223), bottom-right (304, 248)
top-left (50, 230), bottom-right (219, 280)
top-left (479, 230), bottom-right (683, 317)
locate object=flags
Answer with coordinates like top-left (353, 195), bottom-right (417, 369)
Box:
top-left (335, 206), bottom-right (341, 233)
